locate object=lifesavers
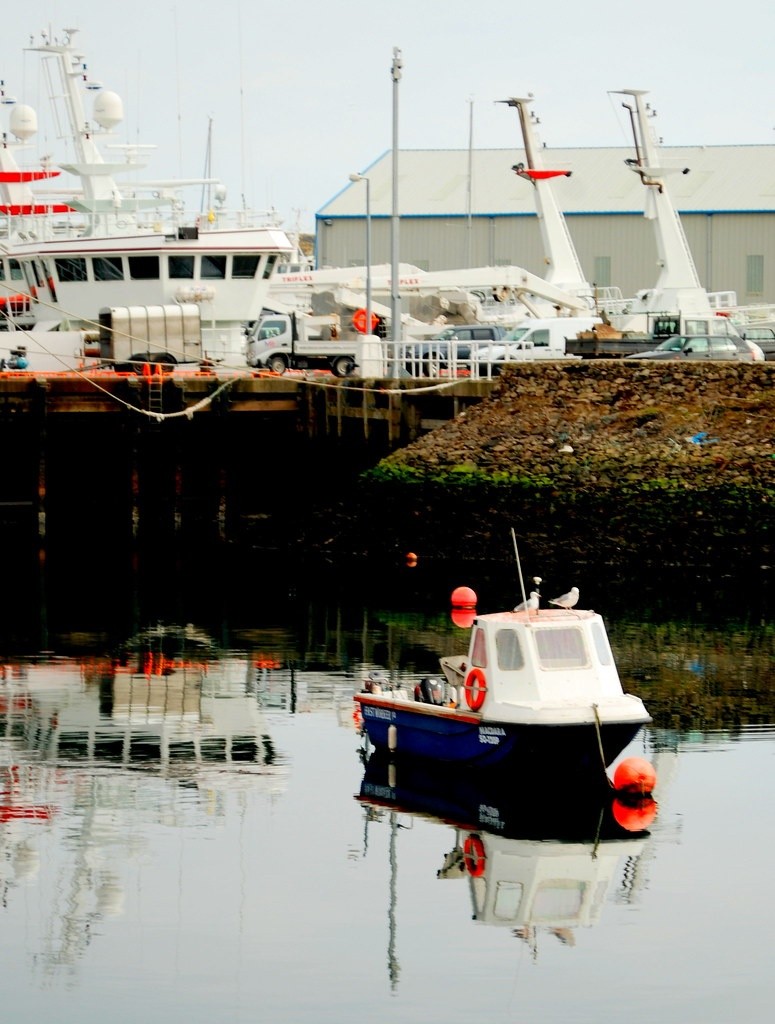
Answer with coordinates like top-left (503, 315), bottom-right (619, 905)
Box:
top-left (354, 308), bottom-right (376, 333)
top-left (464, 667), bottom-right (485, 711)
top-left (463, 833), bottom-right (487, 877)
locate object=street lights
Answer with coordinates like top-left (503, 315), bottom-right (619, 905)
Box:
top-left (350, 174), bottom-right (371, 336)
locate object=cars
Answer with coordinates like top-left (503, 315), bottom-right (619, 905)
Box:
top-left (743, 327), bottom-right (775, 362)
top-left (625, 334), bottom-right (755, 362)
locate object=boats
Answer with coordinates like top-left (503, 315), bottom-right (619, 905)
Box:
top-left (352, 526), bottom-right (651, 795)
top-left (0, 22), bottom-right (775, 370)
top-left (354, 749), bottom-right (652, 991)
top-left (0, 651), bottom-right (286, 984)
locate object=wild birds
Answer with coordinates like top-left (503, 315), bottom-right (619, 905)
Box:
top-left (514, 591), bottom-right (542, 612)
top-left (547, 586), bottom-right (580, 611)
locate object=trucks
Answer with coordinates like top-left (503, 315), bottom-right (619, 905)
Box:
top-left (563, 309), bottom-right (766, 361)
top-left (244, 310), bottom-right (358, 377)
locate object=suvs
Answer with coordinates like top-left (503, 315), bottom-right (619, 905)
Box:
top-left (401, 324), bottom-right (507, 377)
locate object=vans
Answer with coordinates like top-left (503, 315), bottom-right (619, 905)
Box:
top-left (466, 316), bottom-right (603, 377)
